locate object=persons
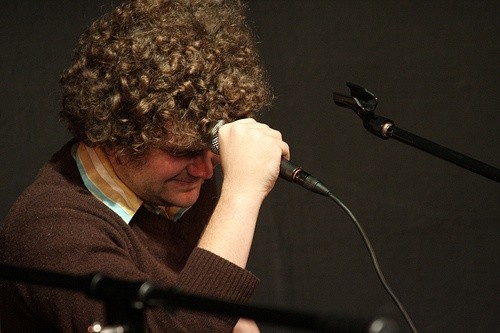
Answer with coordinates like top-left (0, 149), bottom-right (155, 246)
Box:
top-left (0, 0), bottom-right (290, 333)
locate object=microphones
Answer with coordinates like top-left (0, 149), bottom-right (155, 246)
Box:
top-left (207, 122), bottom-right (331, 197)
